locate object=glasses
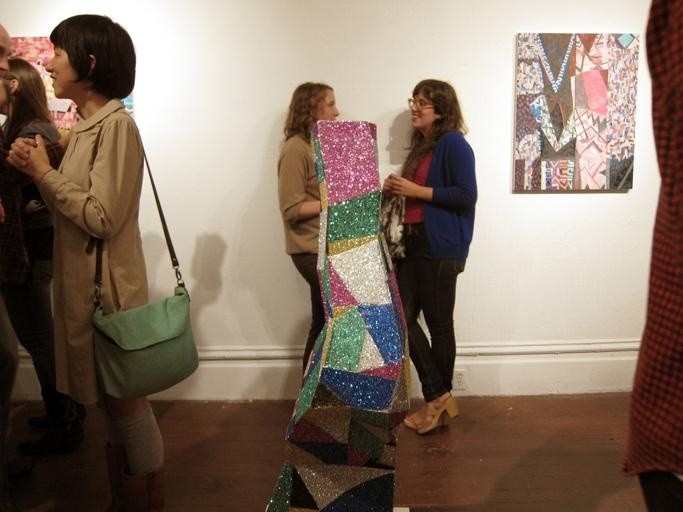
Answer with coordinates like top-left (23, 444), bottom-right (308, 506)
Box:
top-left (409, 97), bottom-right (433, 112)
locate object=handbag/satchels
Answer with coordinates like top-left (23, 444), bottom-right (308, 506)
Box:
top-left (92, 285), bottom-right (199, 401)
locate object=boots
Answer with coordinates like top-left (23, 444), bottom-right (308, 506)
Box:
top-left (102, 441), bottom-right (167, 511)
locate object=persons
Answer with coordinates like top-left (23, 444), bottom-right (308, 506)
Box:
top-left (382, 79), bottom-right (477, 435)
top-left (621, 0), bottom-right (683, 511)
top-left (277, 84), bottom-right (340, 372)
top-left (0, 22), bottom-right (21, 412)
top-left (8, 15), bottom-right (170, 508)
top-left (0, 59), bottom-right (85, 459)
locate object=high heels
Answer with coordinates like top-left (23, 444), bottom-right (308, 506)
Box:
top-left (405, 392), bottom-right (459, 434)
